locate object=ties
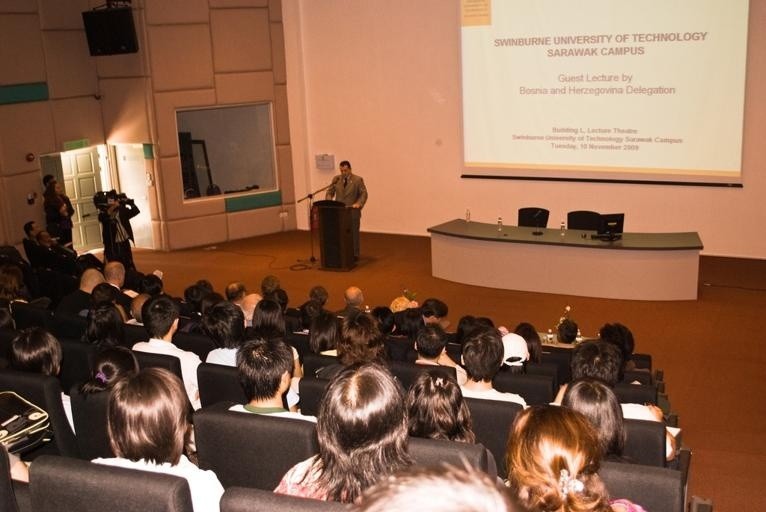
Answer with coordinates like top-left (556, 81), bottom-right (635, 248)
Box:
top-left (597, 212), bottom-right (624, 234)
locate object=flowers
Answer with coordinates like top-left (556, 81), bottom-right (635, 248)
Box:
top-left (556, 305), bottom-right (571, 330)
top-left (533, 210), bottom-right (542, 219)
top-left (334, 177), bottom-right (339, 184)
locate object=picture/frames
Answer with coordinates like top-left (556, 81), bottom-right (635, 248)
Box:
top-left (427, 218), bottom-right (705, 300)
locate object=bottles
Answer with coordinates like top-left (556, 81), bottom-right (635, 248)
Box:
top-left (390, 295), bottom-right (419, 313)
top-left (502, 333), bottom-right (529, 366)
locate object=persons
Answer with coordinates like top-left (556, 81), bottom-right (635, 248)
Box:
top-left (78, 347), bottom-right (139, 398)
top-left (228, 334), bottom-right (318, 424)
top-left (139, 274), bottom-right (163, 301)
top-left (34, 233), bottom-right (77, 272)
top-left (546, 340), bottom-right (677, 462)
top-left (390, 294), bottom-right (418, 313)
top-left (336, 287), bottom-right (363, 319)
top-left (515, 324), bottom-right (543, 366)
top-left (1, 263), bottom-right (29, 305)
top-left (12, 326), bottom-right (77, 436)
top-left (205, 300), bottom-right (301, 413)
top-left (44, 182), bottom-right (73, 251)
top-left (446, 316), bottom-right (475, 342)
top-left (326, 161), bottom-right (368, 262)
top-left (183, 286), bottom-right (207, 314)
top-left (464, 318), bottom-right (495, 338)
top-left (309, 286), bottom-right (332, 314)
top-left (182, 291), bottom-right (224, 334)
top-left (80, 284), bottom-right (128, 324)
top-left (558, 378), bottom-right (639, 466)
top-left (1, 309), bottom-right (18, 347)
top-left (43, 174), bottom-right (74, 222)
top-left (98, 193), bottom-right (139, 274)
top-left (293, 299), bottom-right (322, 334)
top-left (404, 369), bottom-right (478, 445)
top-left (252, 299), bottom-right (304, 380)
top-left (551, 318), bottom-right (578, 350)
top-left (390, 298), bottom-right (450, 346)
top-left (131, 295), bottom-right (206, 454)
top-left (500, 333), bottom-right (530, 377)
top-left (458, 330), bottom-right (531, 409)
top-left (268, 287), bottom-right (299, 333)
top-left (505, 403), bottom-right (645, 512)
top-left (262, 275), bottom-right (279, 295)
top-left (82, 368), bottom-right (224, 512)
top-left (121, 272), bottom-right (144, 299)
top-left (275, 363), bottom-right (412, 506)
top-left (73, 302), bottom-right (122, 350)
top-left (597, 322), bottom-right (637, 370)
top-left (316, 311), bottom-right (407, 397)
top-left (339, 459), bottom-right (513, 512)
top-left (309, 309), bottom-right (343, 357)
top-left (55, 269), bottom-right (104, 318)
top-left (239, 292), bottom-right (262, 328)
top-left (198, 280), bottom-right (213, 294)
top-left (24, 221), bottom-right (44, 247)
top-left (104, 262), bottom-right (139, 301)
top-left (225, 281), bottom-right (246, 306)
top-left (390, 322), bottom-right (468, 391)
top-left (372, 306), bottom-right (408, 362)
top-left (130, 293), bottom-right (152, 327)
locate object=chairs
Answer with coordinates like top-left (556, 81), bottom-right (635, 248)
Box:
top-left (517, 207), bottom-right (550, 230)
top-left (568, 210), bottom-right (602, 231)
top-left (0, 239), bottom-right (714, 512)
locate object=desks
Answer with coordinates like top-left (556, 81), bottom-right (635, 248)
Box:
top-left (427, 218), bottom-right (705, 300)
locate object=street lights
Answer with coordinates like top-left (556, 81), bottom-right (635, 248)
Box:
top-left (0, 391), bottom-right (53, 454)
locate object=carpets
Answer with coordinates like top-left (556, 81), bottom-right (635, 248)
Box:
top-left (344, 177), bottom-right (347, 188)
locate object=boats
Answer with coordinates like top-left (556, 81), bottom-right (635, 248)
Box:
top-left (81, 6), bottom-right (139, 57)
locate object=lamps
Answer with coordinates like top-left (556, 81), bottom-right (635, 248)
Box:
top-left (568, 210), bottom-right (602, 231)
top-left (517, 207), bottom-right (550, 230)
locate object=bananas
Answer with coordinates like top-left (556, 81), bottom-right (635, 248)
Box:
top-left (590, 234), bottom-right (622, 240)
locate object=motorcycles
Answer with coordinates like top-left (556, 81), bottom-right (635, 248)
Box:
top-left (297, 199), bottom-right (320, 267)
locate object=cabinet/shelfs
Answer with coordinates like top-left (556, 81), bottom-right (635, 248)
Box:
top-left (560, 222), bottom-right (566, 237)
top-left (575, 329), bottom-right (583, 345)
top-left (364, 306), bottom-right (371, 313)
top-left (466, 209), bottom-right (470, 222)
top-left (547, 328), bottom-right (553, 345)
top-left (497, 216), bottom-right (502, 232)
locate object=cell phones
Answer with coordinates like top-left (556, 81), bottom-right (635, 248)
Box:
top-left (117, 196), bottom-right (134, 207)
